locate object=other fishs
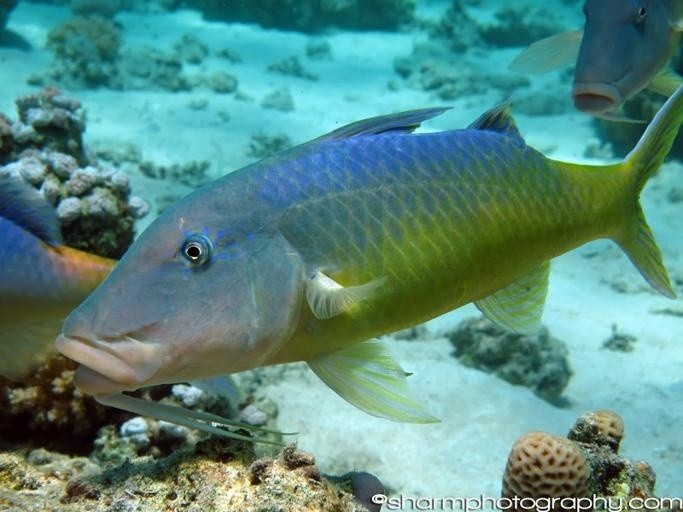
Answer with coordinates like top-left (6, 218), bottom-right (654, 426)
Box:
top-left (54, 82), bottom-right (683, 447)
top-left (0, 169), bottom-right (245, 407)
top-left (506, 0), bottom-right (683, 116)
